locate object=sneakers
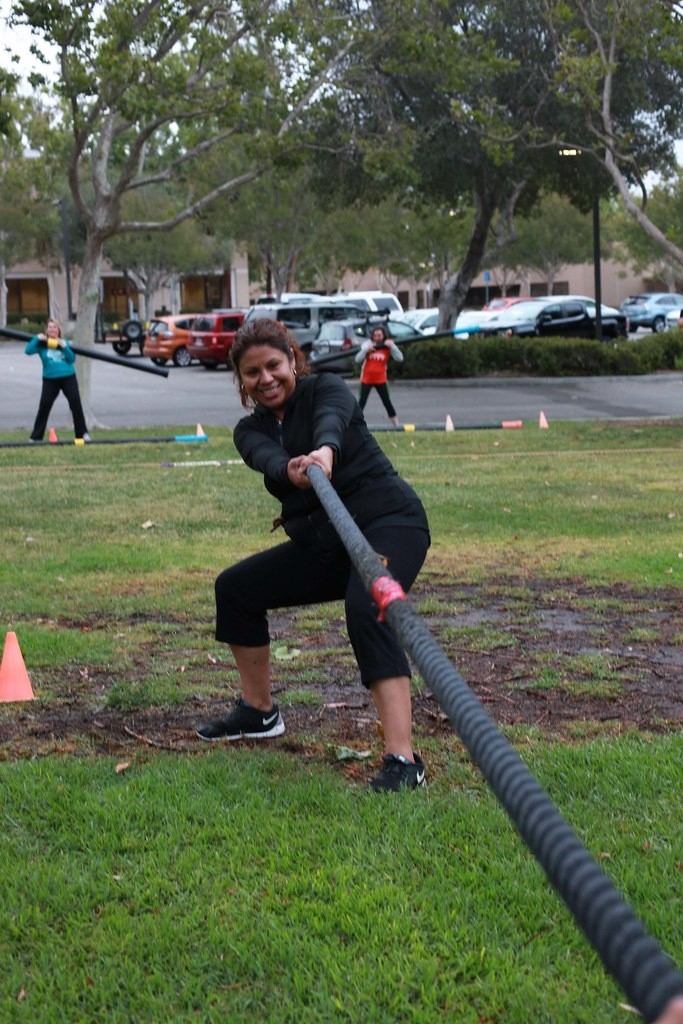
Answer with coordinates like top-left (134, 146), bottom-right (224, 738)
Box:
top-left (196, 697), bottom-right (284, 740)
top-left (371, 750), bottom-right (428, 791)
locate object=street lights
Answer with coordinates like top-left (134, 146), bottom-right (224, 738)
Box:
top-left (559, 146), bottom-right (602, 341)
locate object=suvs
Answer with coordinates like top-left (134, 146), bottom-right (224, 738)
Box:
top-left (249, 291), bottom-right (402, 321)
top-left (620, 292), bottom-right (683, 333)
top-left (245, 302), bottom-right (368, 360)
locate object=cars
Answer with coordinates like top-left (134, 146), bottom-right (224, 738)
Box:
top-left (94, 303), bottom-right (171, 356)
top-left (481, 296), bottom-right (536, 312)
top-left (395, 307), bottom-right (441, 334)
top-left (480, 300), bottom-right (594, 340)
top-left (144, 313), bottom-right (210, 367)
top-left (186, 311), bottom-right (247, 370)
top-left (665, 310), bottom-right (680, 333)
top-left (308, 319), bottom-right (424, 362)
top-left (536, 295), bottom-right (629, 342)
top-left (422, 311), bottom-right (501, 340)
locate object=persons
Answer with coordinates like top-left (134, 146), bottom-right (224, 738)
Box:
top-left (354, 326), bottom-right (403, 427)
top-left (24, 319), bottom-right (91, 442)
top-left (196, 318), bottom-right (433, 793)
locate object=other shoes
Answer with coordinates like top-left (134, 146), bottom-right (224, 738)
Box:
top-left (83, 432), bottom-right (91, 441)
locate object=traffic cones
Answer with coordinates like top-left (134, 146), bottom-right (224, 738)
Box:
top-left (445, 414), bottom-right (455, 432)
top-left (0, 631), bottom-right (35, 704)
top-left (196, 423), bottom-right (204, 437)
top-left (539, 411), bottom-right (549, 429)
top-left (49, 428), bottom-right (57, 442)
